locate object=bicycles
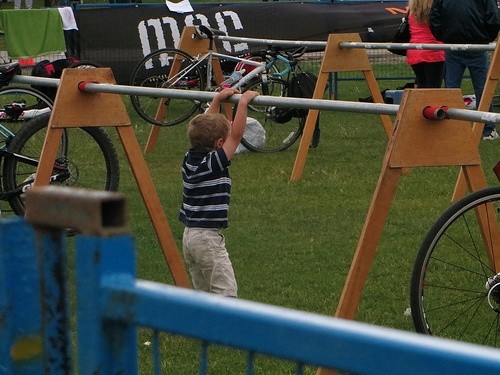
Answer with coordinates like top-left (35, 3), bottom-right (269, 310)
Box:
top-left (131, 25), bottom-right (310, 154)
top-left (408, 108), bottom-right (500, 345)
top-left (0, 108), bottom-right (119, 224)
top-left (0, 64), bottom-right (69, 167)
top-left (235, 43), bottom-right (316, 114)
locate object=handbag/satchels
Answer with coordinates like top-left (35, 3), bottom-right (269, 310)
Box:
top-left (288, 64), bottom-right (329, 118)
top-left (30, 55), bottom-right (79, 105)
top-left (387, 9), bottom-right (411, 56)
top-left (266, 106), bottom-right (292, 124)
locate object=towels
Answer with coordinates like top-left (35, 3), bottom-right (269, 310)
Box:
top-left (58, 4), bottom-right (81, 31)
top-left (4, 9), bottom-right (79, 58)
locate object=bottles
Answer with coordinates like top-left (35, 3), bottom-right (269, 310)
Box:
top-left (220, 69), bottom-right (246, 89)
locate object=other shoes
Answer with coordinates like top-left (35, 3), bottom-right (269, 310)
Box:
top-left (482, 129), bottom-right (499, 141)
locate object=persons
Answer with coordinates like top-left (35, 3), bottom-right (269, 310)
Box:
top-left (428, 0), bottom-right (500, 140)
top-left (405, 0), bottom-right (446, 87)
top-left (177, 88), bottom-right (260, 298)
